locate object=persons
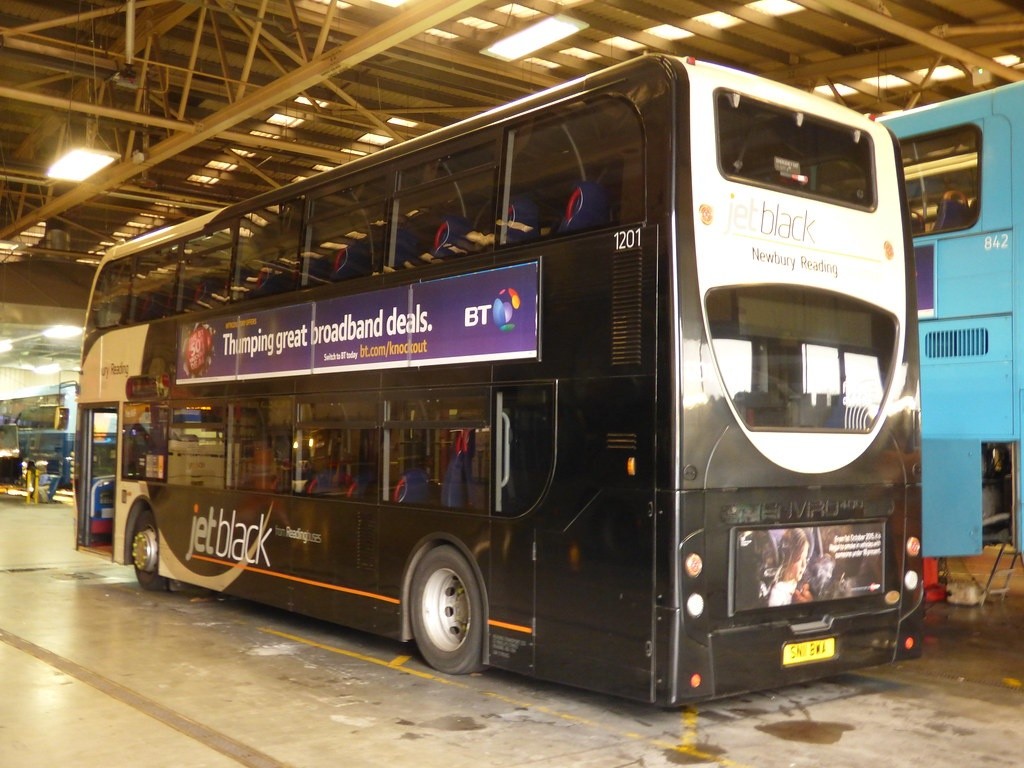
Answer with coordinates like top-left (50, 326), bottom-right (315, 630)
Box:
top-left (738, 523), bottom-right (874, 611)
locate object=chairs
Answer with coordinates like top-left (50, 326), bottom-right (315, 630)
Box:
top-left (910, 190), bottom-right (978, 236)
top-left (118, 178), bottom-right (615, 326)
top-left (304, 427), bottom-right (478, 509)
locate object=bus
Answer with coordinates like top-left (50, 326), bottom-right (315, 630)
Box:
top-left (75, 54), bottom-right (923, 712)
top-left (871, 79), bottom-right (1023, 600)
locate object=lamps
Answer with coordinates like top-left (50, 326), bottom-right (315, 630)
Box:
top-left (479, 1), bottom-right (590, 62)
top-left (46, 1), bottom-right (121, 183)
